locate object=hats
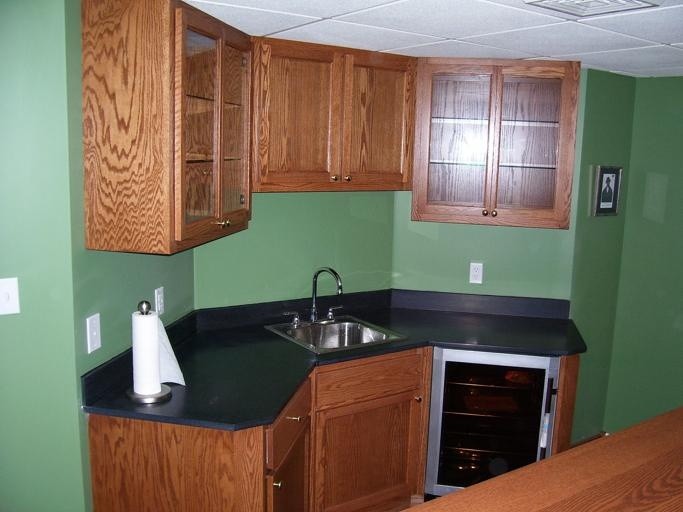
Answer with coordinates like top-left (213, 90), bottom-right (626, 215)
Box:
top-left (605, 177), bottom-right (611, 183)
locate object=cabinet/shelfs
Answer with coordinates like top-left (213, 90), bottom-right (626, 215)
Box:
top-left (82, 373), bottom-right (315, 512)
top-left (310, 342), bottom-right (429, 512)
top-left (78, 1), bottom-right (251, 259)
top-left (407, 54), bottom-right (581, 233)
top-left (250, 32), bottom-right (417, 196)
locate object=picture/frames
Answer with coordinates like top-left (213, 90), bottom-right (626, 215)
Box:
top-left (593, 163), bottom-right (621, 219)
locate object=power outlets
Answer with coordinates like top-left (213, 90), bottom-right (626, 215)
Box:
top-left (468, 261), bottom-right (483, 285)
top-left (152, 285), bottom-right (165, 316)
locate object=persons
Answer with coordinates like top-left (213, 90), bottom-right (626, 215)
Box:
top-left (601, 177), bottom-right (614, 202)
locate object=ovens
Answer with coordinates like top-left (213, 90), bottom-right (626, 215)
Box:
top-left (422, 344), bottom-right (562, 497)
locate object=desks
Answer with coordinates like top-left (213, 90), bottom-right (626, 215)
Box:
top-left (403, 405), bottom-right (681, 511)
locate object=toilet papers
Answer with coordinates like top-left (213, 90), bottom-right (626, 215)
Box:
top-left (131, 310), bottom-right (186, 396)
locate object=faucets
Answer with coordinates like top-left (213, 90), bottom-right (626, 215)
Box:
top-left (309, 266), bottom-right (344, 324)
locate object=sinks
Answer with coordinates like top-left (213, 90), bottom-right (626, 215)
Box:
top-left (263, 313), bottom-right (410, 355)
